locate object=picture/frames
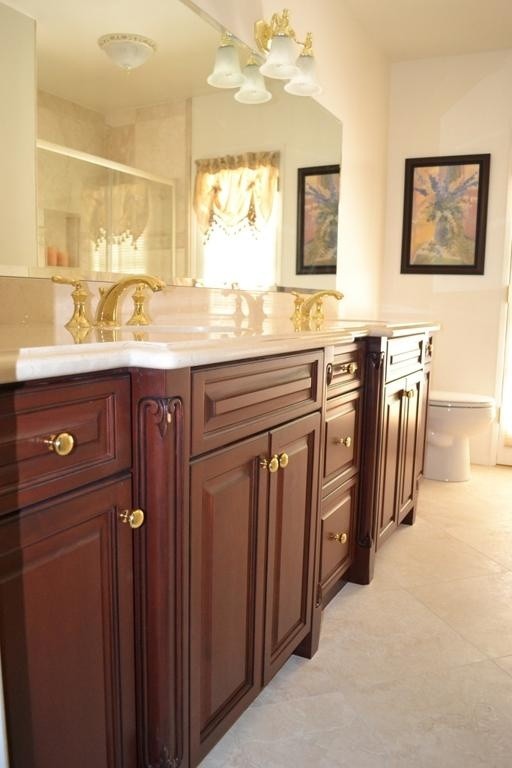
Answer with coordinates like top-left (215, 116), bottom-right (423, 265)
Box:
top-left (398, 153), bottom-right (492, 274)
top-left (295, 164), bottom-right (342, 275)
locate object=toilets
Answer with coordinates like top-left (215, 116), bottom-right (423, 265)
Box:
top-left (424, 392), bottom-right (496, 480)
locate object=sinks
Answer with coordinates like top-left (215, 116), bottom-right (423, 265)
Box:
top-left (110, 324), bottom-right (206, 335)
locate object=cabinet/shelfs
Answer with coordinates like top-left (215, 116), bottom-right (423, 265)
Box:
top-left (351, 331), bottom-right (441, 586)
top-left (3, 369), bottom-right (130, 768)
top-left (318, 351), bottom-right (367, 612)
top-left (131, 349), bottom-right (321, 768)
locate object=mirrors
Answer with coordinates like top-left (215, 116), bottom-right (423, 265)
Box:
top-left (0, 0), bottom-right (346, 298)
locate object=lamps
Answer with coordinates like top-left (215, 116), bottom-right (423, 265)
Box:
top-left (205, 30), bottom-right (273, 106)
top-left (99, 33), bottom-right (158, 71)
top-left (254, 9), bottom-right (317, 105)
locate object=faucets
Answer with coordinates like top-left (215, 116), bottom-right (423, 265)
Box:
top-left (301, 290), bottom-right (343, 329)
top-left (97, 275), bottom-right (165, 323)
top-left (223, 288), bottom-right (258, 331)
